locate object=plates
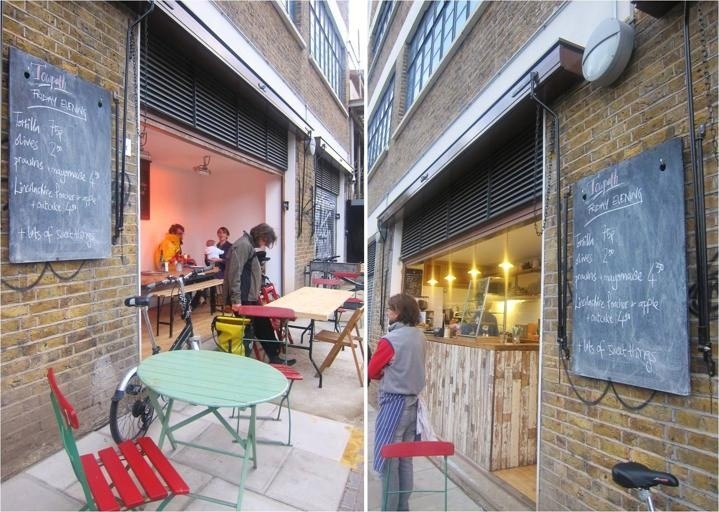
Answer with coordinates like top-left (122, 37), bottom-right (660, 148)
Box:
top-left (141, 271), bottom-right (163, 276)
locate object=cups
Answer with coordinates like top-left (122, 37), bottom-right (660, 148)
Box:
top-left (515, 324), bottom-right (529, 339)
top-left (443, 322), bottom-right (450, 339)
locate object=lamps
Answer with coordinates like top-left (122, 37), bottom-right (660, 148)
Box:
top-left (192, 154), bottom-right (212, 176)
top-left (139, 130), bottom-right (151, 160)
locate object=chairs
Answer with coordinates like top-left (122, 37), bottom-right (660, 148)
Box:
top-left (254, 282), bottom-right (294, 360)
top-left (47, 367), bottom-right (189, 511)
top-left (230, 305), bottom-right (304, 446)
top-left (300, 271), bottom-right (364, 351)
top-left (314, 304), bottom-right (364, 388)
top-left (380, 441), bottom-right (455, 511)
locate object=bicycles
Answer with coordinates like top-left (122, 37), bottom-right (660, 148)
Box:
top-left (108, 264), bottom-right (216, 443)
top-left (613, 461), bottom-right (680, 512)
top-left (310, 255), bottom-right (365, 292)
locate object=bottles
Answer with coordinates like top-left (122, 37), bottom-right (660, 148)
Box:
top-left (159, 249), bottom-right (169, 273)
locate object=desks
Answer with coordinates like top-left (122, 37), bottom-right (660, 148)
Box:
top-left (135, 350), bottom-right (289, 512)
top-left (265, 286), bottom-right (355, 389)
top-left (418, 336), bottom-right (539, 472)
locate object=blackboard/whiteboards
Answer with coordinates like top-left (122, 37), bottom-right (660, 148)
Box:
top-left (568, 137), bottom-right (692, 397)
top-left (8, 45), bottom-right (113, 264)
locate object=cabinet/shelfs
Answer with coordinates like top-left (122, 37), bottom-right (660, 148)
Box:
top-left (424, 260), bottom-right (503, 291)
top-left (506, 266), bottom-right (541, 300)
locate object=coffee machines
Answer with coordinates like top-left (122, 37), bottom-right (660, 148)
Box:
top-left (414, 296), bottom-right (429, 325)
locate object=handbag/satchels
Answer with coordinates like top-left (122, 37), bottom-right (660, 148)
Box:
top-left (211, 310), bottom-right (255, 356)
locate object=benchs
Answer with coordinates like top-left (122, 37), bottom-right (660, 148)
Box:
top-left (150, 278), bottom-right (224, 338)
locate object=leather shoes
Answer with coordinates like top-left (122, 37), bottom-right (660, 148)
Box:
top-left (269, 358), bottom-right (296, 366)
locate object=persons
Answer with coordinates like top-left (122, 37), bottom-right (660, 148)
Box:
top-left (469, 293), bottom-right (498, 336)
top-left (204, 240), bottom-right (225, 267)
top-left (223, 223), bottom-right (296, 366)
top-left (368, 294), bottom-right (427, 512)
top-left (153, 224), bottom-right (206, 305)
top-left (205, 227), bottom-right (233, 315)
